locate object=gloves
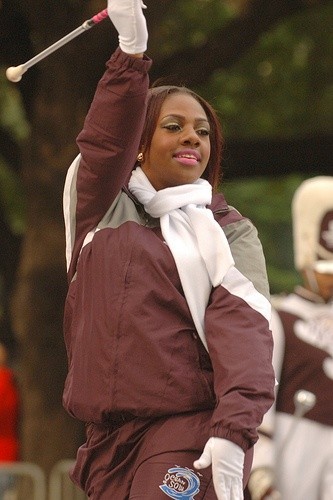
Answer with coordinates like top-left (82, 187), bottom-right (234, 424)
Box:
top-left (194, 437), bottom-right (244, 500)
top-left (107, 0), bottom-right (148, 54)
top-left (247, 467), bottom-right (281, 500)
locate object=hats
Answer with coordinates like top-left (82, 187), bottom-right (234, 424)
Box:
top-left (291, 176), bottom-right (333, 275)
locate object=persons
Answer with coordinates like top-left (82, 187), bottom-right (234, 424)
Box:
top-left (248, 175), bottom-right (333, 500)
top-left (63, 0), bottom-right (276, 500)
